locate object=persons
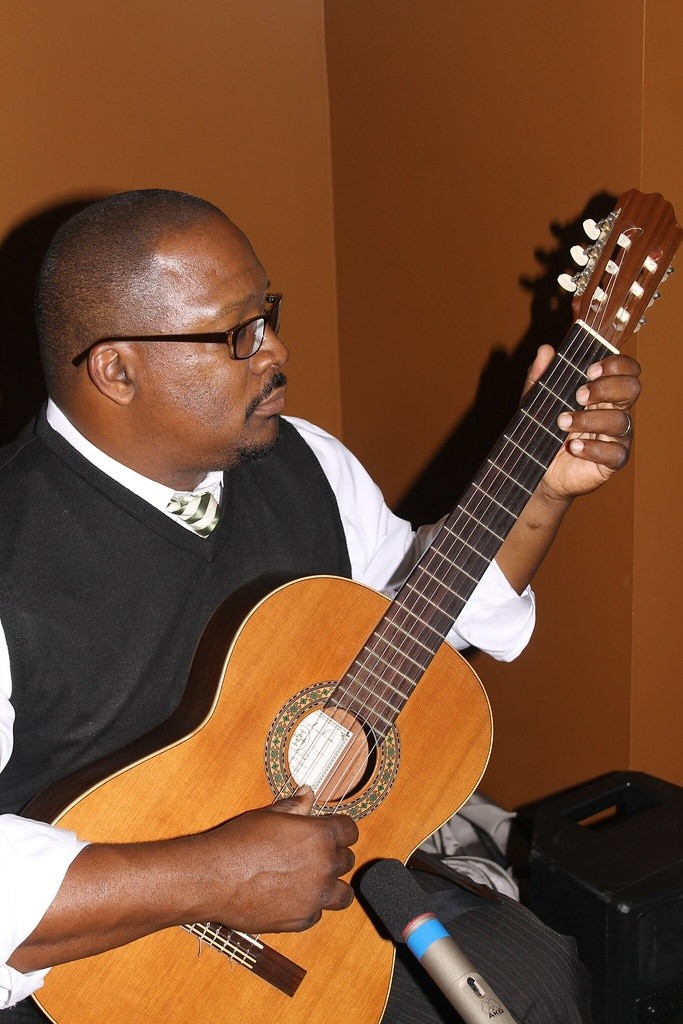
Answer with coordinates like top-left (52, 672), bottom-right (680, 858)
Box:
top-left (2, 185), bottom-right (643, 1022)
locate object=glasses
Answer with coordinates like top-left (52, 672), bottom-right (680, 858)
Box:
top-left (73, 292), bottom-right (286, 368)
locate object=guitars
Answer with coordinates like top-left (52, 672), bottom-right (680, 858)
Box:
top-left (28, 188), bottom-right (682, 1024)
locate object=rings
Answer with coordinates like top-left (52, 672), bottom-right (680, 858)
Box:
top-left (617, 412), bottom-right (634, 439)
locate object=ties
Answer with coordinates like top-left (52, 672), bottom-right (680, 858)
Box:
top-left (165, 490), bottom-right (222, 537)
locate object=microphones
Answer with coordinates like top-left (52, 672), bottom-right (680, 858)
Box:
top-left (362, 857), bottom-right (519, 1024)
